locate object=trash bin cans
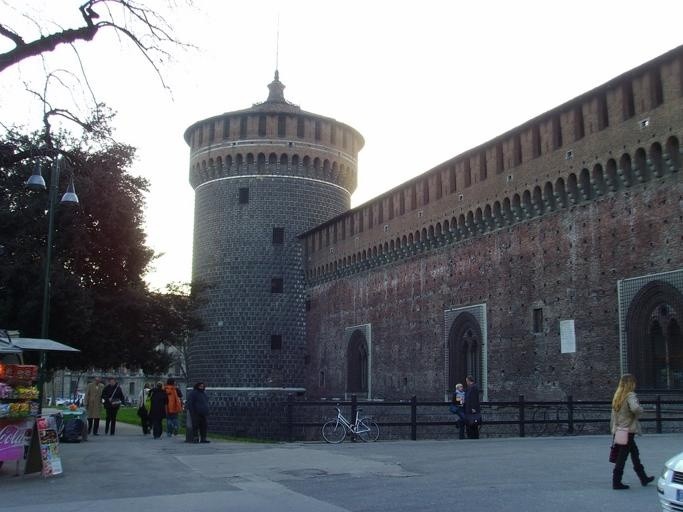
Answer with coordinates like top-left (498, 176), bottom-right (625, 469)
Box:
top-left (63, 409), bottom-right (83, 425)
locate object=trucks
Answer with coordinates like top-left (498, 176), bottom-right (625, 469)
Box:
top-left (0, 329), bottom-right (79, 365)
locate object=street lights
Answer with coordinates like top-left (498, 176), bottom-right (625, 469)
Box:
top-left (24, 139), bottom-right (79, 419)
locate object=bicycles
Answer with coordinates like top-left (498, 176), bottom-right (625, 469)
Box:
top-left (320, 402), bottom-right (380, 444)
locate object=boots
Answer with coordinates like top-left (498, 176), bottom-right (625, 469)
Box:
top-left (633, 464), bottom-right (653, 486)
top-left (612, 470), bottom-right (627, 489)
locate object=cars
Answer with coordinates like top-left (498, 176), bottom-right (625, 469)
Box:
top-left (655, 451), bottom-right (682, 512)
top-left (47, 392), bottom-right (138, 407)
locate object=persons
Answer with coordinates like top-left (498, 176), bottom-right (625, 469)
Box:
top-left (465, 375), bottom-right (481, 439)
top-left (162, 378), bottom-right (183, 437)
top-left (101, 379), bottom-right (125, 435)
top-left (84, 378), bottom-right (105, 436)
top-left (610, 374), bottom-right (655, 490)
top-left (140, 384), bottom-right (151, 434)
top-left (452, 384), bottom-right (466, 439)
top-left (148, 382), bottom-right (168, 439)
top-left (187, 382), bottom-right (212, 444)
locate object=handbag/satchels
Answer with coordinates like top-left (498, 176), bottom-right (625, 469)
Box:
top-left (614, 428), bottom-right (627, 445)
top-left (449, 404), bottom-right (459, 413)
top-left (608, 447), bottom-right (617, 463)
top-left (467, 412), bottom-right (481, 425)
top-left (111, 401), bottom-right (121, 410)
top-left (137, 407), bottom-right (147, 417)
top-left (185, 400), bottom-right (194, 412)
